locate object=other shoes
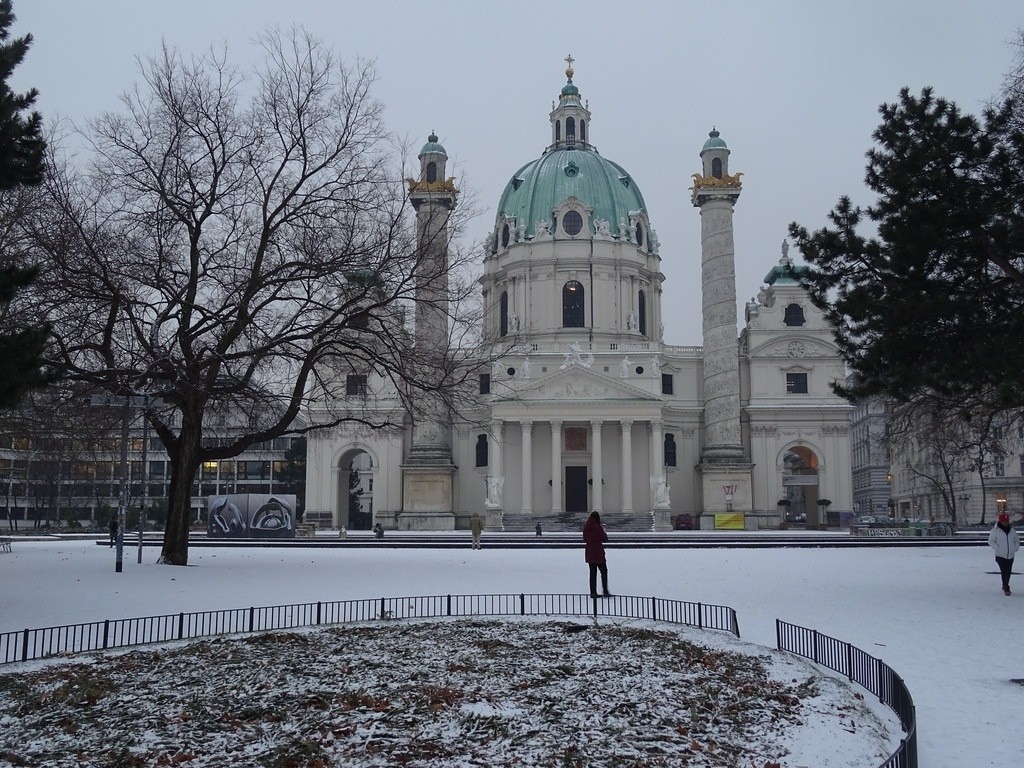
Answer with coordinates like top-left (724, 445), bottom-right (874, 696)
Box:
top-left (1002, 587), bottom-right (1011, 595)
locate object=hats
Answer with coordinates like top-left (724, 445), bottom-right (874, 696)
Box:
top-left (999, 514), bottom-right (1009, 523)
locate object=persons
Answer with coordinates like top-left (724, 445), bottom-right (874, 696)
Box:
top-left (535, 522), bottom-right (542, 538)
top-left (987, 513), bottom-right (1020, 596)
top-left (108, 516), bottom-right (118, 548)
top-left (373, 523), bottom-right (384, 539)
top-left (470, 513), bottom-right (484, 550)
top-left (583, 511), bottom-right (613, 597)
top-left (339, 525), bottom-right (347, 538)
